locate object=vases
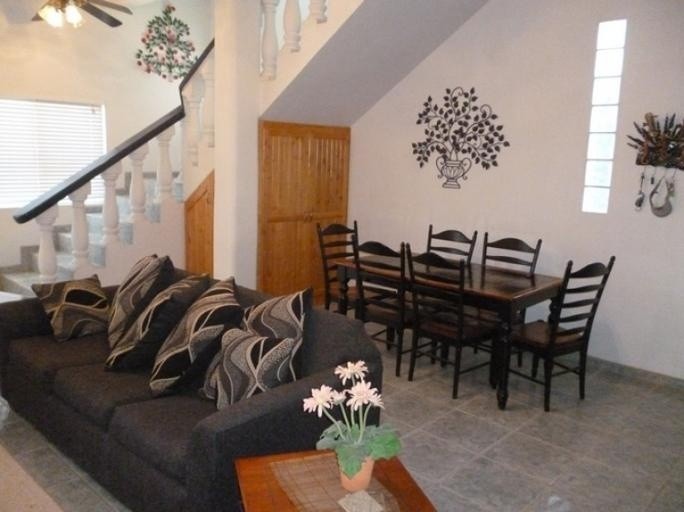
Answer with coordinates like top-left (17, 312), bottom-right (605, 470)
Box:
top-left (336, 455), bottom-right (377, 492)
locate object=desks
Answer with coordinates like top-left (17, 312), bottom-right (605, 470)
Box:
top-left (325, 250), bottom-right (564, 412)
top-left (227, 448), bottom-right (435, 511)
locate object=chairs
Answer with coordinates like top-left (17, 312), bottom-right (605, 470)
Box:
top-left (399, 224), bottom-right (480, 365)
top-left (505, 252), bottom-right (615, 411)
top-left (350, 232), bottom-right (439, 380)
top-left (402, 241), bottom-right (501, 401)
top-left (464, 232), bottom-right (543, 368)
top-left (313, 220), bottom-right (394, 353)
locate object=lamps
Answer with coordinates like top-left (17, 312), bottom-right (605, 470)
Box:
top-left (38, 2), bottom-right (84, 29)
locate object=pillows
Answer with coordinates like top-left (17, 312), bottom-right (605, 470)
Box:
top-left (102, 270), bottom-right (210, 377)
top-left (30, 273), bottom-right (110, 344)
top-left (106, 250), bottom-right (175, 354)
top-left (195, 286), bottom-right (312, 399)
top-left (212, 323), bottom-right (298, 411)
top-left (148, 276), bottom-right (243, 399)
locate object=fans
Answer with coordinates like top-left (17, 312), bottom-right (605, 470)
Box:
top-left (32, 1), bottom-right (133, 29)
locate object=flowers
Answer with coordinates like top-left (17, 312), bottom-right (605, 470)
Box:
top-left (304, 359), bottom-right (403, 475)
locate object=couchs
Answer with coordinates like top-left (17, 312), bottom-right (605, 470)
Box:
top-left (1, 265), bottom-right (382, 511)
